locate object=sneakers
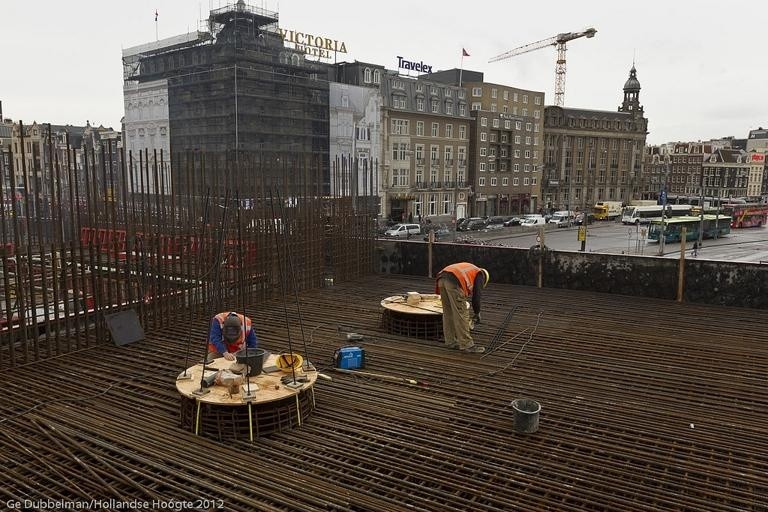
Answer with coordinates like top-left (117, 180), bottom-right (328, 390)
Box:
top-left (448, 343), bottom-right (460, 349)
top-left (465, 345), bottom-right (485, 353)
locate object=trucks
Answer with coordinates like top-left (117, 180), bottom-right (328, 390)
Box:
top-left (521, 218), bottom-right (546, 226)
top-left (519, 215), bottom-right (542, 224)
top-left (593, 202), bottom-right (622, 221)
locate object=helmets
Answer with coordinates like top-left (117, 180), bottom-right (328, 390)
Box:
top-left (481, 268), bottom-right (489, 289)
top-left (276, 352), bottom-right (304, 373)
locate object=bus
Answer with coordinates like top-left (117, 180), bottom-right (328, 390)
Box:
top-left (648, 215), bottom-right (732, 243)
top-left (622, 205), bottom-right (692, 225)
top-left (722, 203), bottom-right (767, 228)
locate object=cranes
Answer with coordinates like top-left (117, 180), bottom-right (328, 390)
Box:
top-left (487, 27), bottom-right (599, 107)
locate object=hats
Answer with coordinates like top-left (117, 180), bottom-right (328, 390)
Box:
top-left (222, 316), bottom-right (241, 338)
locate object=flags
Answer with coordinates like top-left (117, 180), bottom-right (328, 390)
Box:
top-left (462, 48), bottom-right (471, 56)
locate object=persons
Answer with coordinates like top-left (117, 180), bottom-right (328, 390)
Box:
top-left (690, 242), bottom-right (698, 256)
top-left (418, 214), bottom-right (431, 223)
top-left (435, 262), bottom-right (490, 353)
top-left (206, 311), bottom-right (257, 360)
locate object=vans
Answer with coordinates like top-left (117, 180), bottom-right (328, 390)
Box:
top-left (458, 218), bottom-right (487, 231)
top-left (548, 210), bottom-right (575, 227)
top-left (385, 223), bottom-right (421, 237)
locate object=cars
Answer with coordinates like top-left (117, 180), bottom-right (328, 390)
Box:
top-left (486, 216), bottom-right (504, 224)
top-left (456, 218), bottom-right (466, 230)
top-left (504, 217), bottom-right (520, 226)
top-left (575, 214), bottom-right (592, 225)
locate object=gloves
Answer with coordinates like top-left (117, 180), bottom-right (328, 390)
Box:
top-left (472, 313), bottom-right (481, 324)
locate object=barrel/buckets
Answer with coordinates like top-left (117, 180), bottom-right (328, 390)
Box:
top-left (236, 348), bottom-right (267, 376)
top-left (510, 395), bottom-right (544, 434)
